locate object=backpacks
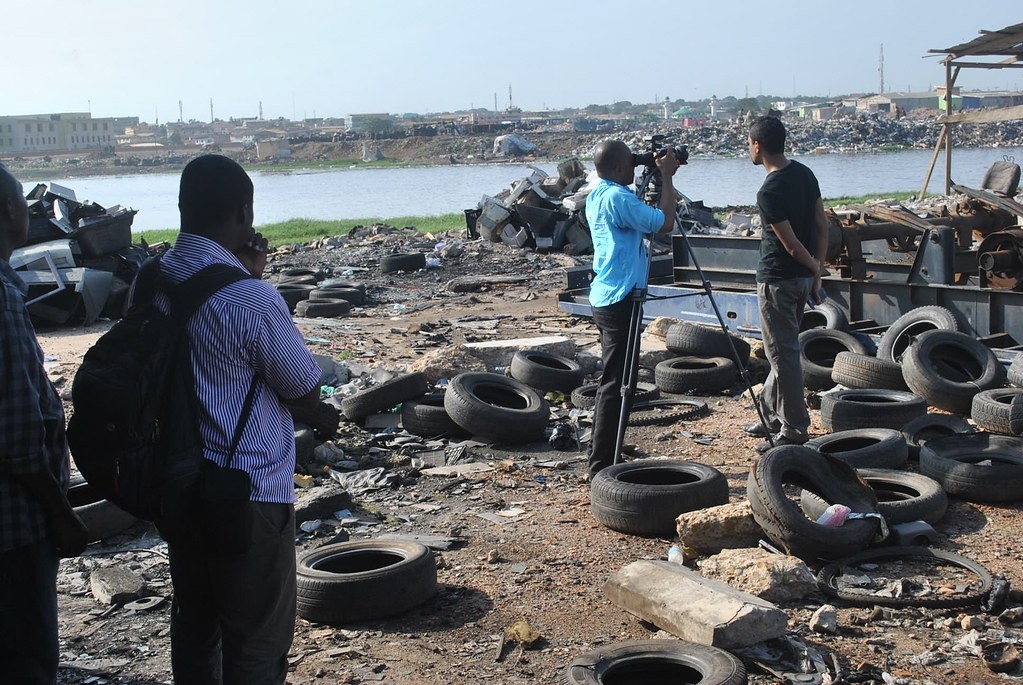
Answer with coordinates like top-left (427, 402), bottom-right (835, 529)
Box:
top-left (67, 253), bottom-right (261, 521)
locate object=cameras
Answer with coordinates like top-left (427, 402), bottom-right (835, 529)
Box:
top-left (632, 135), bottom-right (689, 167)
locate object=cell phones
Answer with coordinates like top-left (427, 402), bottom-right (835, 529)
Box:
top-left (807, 287), bottom-right (828, 310)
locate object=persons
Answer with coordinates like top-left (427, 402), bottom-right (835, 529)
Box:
top-left (1, 164), bottom-right (89, 685)
top-left (126, 153), bottom-right (343, 683)
top-left (585, 140), bottom-right (679, 484)
top-left (743, 115), bottom-right (829, 453)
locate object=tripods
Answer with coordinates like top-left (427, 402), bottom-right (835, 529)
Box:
top-left (614, 172), bottom-right (775, 464)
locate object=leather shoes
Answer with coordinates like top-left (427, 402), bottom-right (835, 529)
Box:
top-left (755, 432), bottom-right (809, 455)
top-left (742, 421), bottom-right (780, 436)
